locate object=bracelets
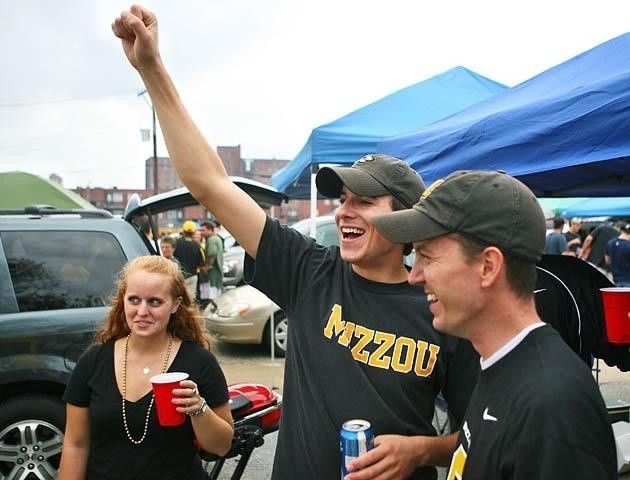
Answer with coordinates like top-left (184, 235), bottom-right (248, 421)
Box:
top-left (183, 395), bottom-right (209, 417)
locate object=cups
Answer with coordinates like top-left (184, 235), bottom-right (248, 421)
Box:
top-left (599, 287), bottom-right (630, 344)
top-left (150, 371), bottom-right (191, 426)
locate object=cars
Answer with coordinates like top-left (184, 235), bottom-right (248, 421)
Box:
top-left (202, 284), bottom-right (287, 358)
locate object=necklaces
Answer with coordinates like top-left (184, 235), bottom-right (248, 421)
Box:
top-left (126, 337), bottom-right (165, 376)
top-left (118, 328), bottom-right (174, 444)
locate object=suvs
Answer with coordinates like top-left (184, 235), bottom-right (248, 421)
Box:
top-left (215, 216), bottom-right (342, 287)
top-left (0, 177), bottom-right (167, 480)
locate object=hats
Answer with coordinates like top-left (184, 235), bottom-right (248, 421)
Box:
top-left (370, 171), bottom-right (546, 262)
top-left (183, 220), bottom-right (196, 233)
top-left (571, 218), bottom-right (583, 224)
top-left (315, 153), bottom-right (426, 208)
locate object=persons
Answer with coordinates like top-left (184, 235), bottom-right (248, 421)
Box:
top-left (110, 3), bottom-right (482, 480)
top-left (545, 217), bottom-right (629, 287)
top-left (135, 213), bottom-right (224, 304)
top-left (367, 166), bottom-right (621, 480)
top-left (55, 251), bottom-right (237, 480)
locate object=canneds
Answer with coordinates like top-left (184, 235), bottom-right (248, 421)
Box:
top-left (339, 419), bottom-right (375, 480)
